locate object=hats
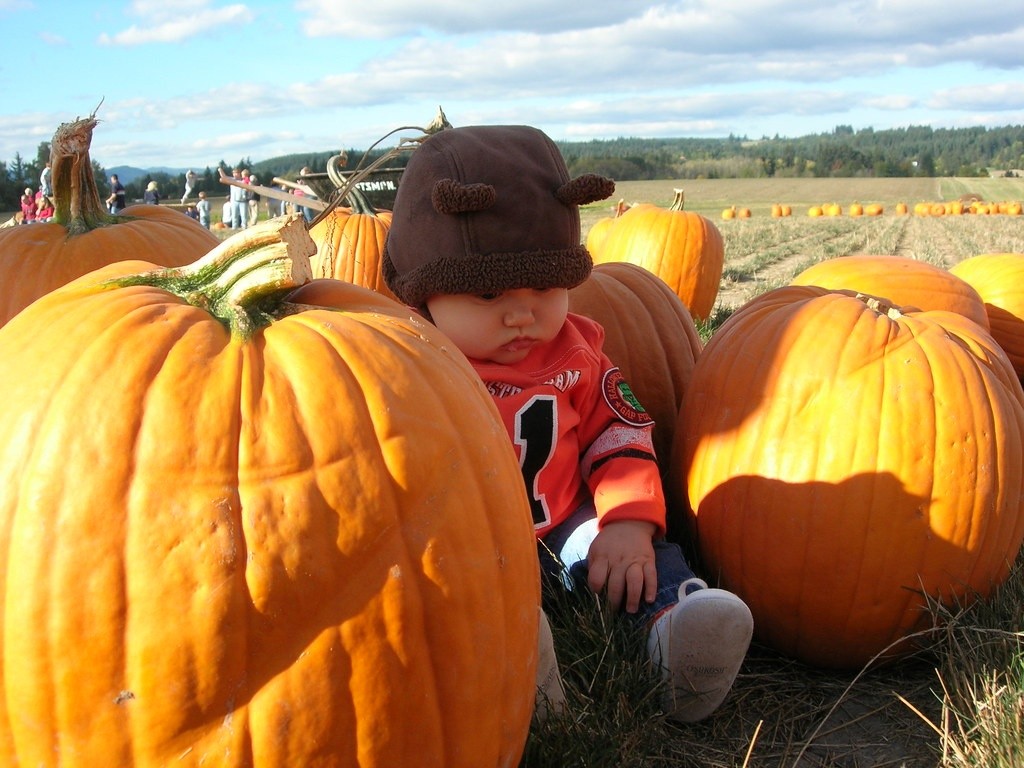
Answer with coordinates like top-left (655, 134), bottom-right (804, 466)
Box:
top-left (383, 125), bottom-right (615, 309)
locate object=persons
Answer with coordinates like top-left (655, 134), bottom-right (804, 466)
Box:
top-left (223, 167), bottom-right (318, 228)
top-left (143, 180), bottom-right (161, 205)
top-left (105, 174), bottom-right (126, 214)
top-left (195, 191), bottom-right (211, 230)
top-left (20, 163), bottom-right (55, 224)
top-left (181, 170), bottom-right (197, 204)
top-left (378, 125), bottom-right (754, 732)
top-left (184, 206), bottom-right (199, 220)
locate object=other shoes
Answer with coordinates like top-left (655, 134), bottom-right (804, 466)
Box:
top-left (646, 578), bottom-right (754, 723)
top-left (530, 608), bottom-right (567, 727)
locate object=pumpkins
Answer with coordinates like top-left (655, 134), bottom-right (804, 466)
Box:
top-left (567, 189), bottom-right (1024, 671)
top-left (0, 118), bottom-right (541, 768)
